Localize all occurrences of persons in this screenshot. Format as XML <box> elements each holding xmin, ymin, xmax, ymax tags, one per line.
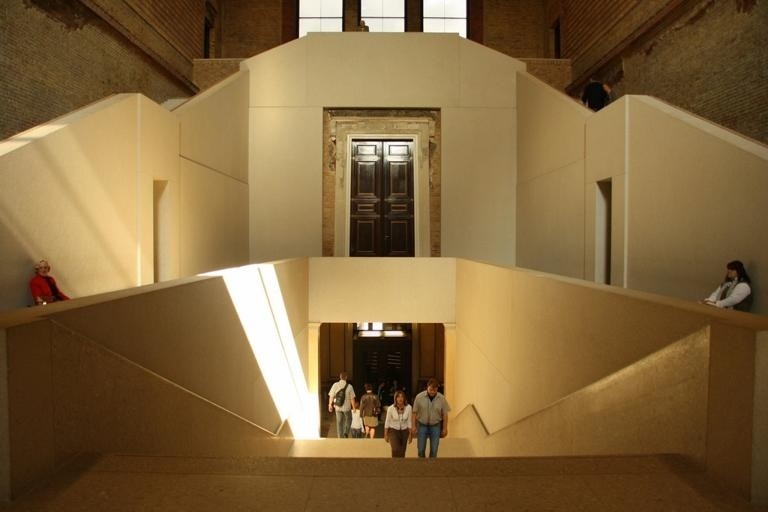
<box><xmin>704</xmin><ymin>260</ymin><xmax>753</xmax><ymax>312</ymax></box>
<box><xmin>328</xmin><ymin>371</ymin><xmax>405</xmax><ymax>439</ymax></box>
<box><xmin>412</xmin><ymin>378</ymin><xmax>451</xmax><ymax>458</ymax></box>
<box><xmin>356</xmin><ymin>21</ymin><xmax>369</xmax><ymax>32</ymax></box>
<box><xmin>29</xmin><ymin>258</ymin><xmax>70</xmax><ymax>306</ymax></box>
<box><xmin>581</xmin><ymin>74</ymin><xmax>610</xmax><ymax>112</ymax></box>
<box><xmin>384</xmin><ymin>391</ymin><xmax>412</xmax><ymax>457</ymax></box>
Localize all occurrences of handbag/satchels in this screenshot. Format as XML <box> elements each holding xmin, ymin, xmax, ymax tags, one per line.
<box><xmin>372</xmin><ymin>406</ymin><xmax>380</xmax><ymax>416</ymax></box>
<box><xmin>332</xmin><ymin>389</ymin><xmax>345</xmax><ymax>406</ymax></box>
